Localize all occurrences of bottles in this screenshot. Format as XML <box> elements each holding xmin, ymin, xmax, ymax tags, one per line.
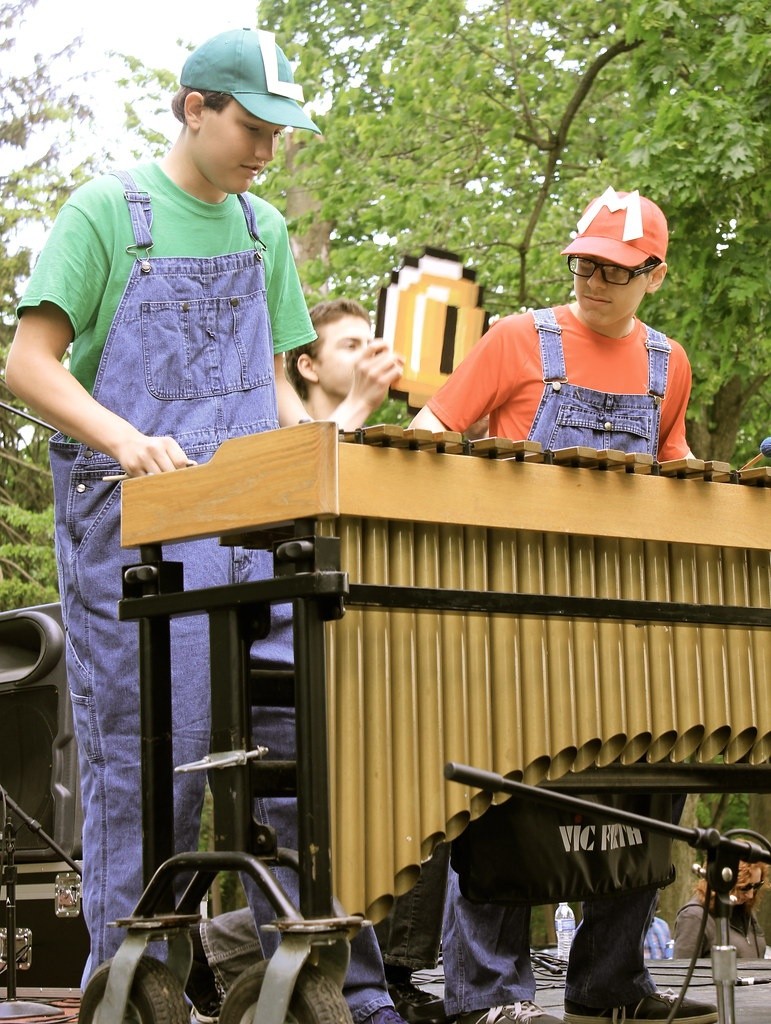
<box><xmin>554</xmin><ymin>903</ymin><xmax>575</xmax><ymax>961</ymax></box>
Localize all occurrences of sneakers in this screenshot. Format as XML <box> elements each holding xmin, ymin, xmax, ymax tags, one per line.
<box><xmin>363</xmin><ymin>1007</ymin><xmax>407</xmax><ymax>1024</ymax></box>
<box><xmin>564</xmin><ymin>987</ymin><xmax>719</xmax><ymax>1024</ymax></box>
<box><xmin>184</xmin><ymin>947</ymin><xmax>220</xmax><ymax>1023</ymax></box>
<box><xmin>387</xmin><ymin>982</ymin><xmax>451</xmax><ymax>1023</ymax></box>
<box><xmin>459</xmin><ymin>999</ymin><xmax>563</xmax><ymax>1024</ymax></box>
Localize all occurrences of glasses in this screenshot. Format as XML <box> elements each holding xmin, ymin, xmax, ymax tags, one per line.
<box><xmin>567</xmin><ymin>260</ymin><xmax>662</xmax><ymax>285</ymax></box>
<box><xmin>738</xmin><ymin>879</ymin><xmax>765</xmax><ymax>891</ymax></box>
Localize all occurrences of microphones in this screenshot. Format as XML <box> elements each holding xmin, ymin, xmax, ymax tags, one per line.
<box><xmin>760</xmin><ymin>437</ymin><xmax>771</xmax><ymax>458</ymax></box>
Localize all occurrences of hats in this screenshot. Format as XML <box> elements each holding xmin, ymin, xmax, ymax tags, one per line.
<box><xmin>181</xmin><ymin>27</ymin><xmax>322</xmax><ymax>136</ymax></box>
<box><xmin>560</xmin><ymin>185</ymin><xmax>668</xmax><ymax>265</ymax></box>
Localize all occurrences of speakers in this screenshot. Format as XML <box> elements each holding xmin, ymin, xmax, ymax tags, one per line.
<box><xmin>0</xmin><ymin>601</ymin><xmax>84</xmax><ymax>863</ymax></box>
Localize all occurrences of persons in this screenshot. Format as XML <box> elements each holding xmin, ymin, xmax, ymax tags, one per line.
<box><xmin>287</xmin><ymin>296</ymin><xmax>450</xmax><ymax>1024</ymax></box>
<box><xmin>6</xmin><ymin>28</ymin><xmax>406</xmax><ymax>1024</ymax></box>
<box><xmin>407</xmin><ymin>188</ymin><xmax>718</xmax><ymax>1024</ymax></box>
<box><xmin>644</xmin><ymin>838</ymin><xmax>768</xmax><ymax>960</ymax></box>
